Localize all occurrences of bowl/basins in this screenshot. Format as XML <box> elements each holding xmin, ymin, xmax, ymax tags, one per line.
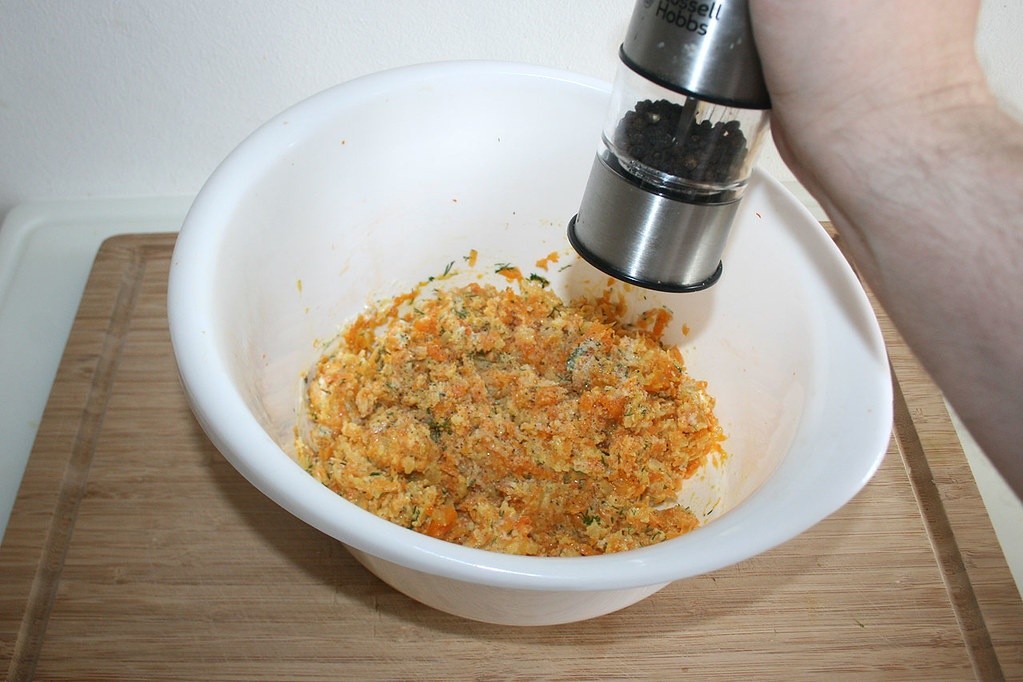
<box><xmin>169</xmin><ymin>54</ymin><xmax>893</xmax><ymax>631</ymax></box>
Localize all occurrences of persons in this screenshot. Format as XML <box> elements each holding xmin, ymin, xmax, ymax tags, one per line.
<box><xmin>750</xmin><ymin>0</ymin><xmax>1022</xmax><ymax>498</ymax></box>
